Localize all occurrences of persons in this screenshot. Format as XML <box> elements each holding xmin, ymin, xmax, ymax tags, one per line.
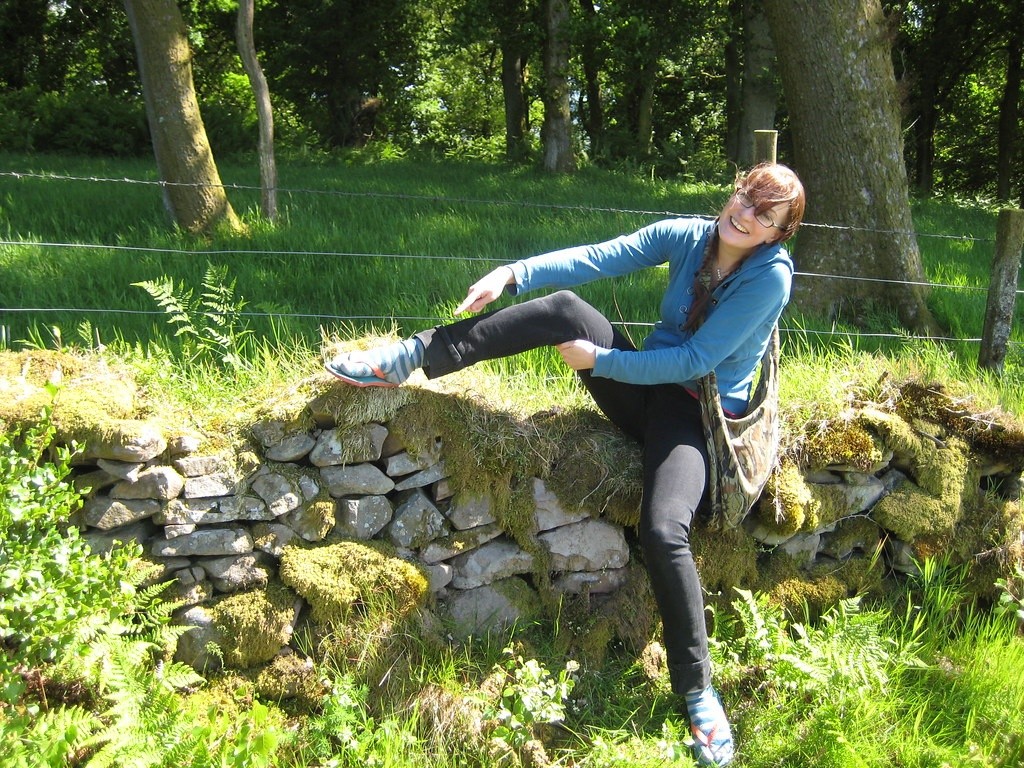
<box><xmin>325</xmin><ymin>162</ymin><xmax>805</xmax><ymax>768</ymax></box>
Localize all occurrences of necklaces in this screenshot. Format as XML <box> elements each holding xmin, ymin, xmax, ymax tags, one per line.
<box><xmin>716</xmin><ymin>264</ymin><xmax>723</xmax><ymax>280</ymax></box>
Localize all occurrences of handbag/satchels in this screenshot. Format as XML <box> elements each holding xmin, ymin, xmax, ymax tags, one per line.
<box><xmin>700</xmin><ymin>373</ymin><xmax>779</xmax><ymax>537</ymax></box>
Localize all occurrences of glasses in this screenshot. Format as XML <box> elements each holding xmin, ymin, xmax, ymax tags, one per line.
<box><xmin>735</xmin><ymin>187</ymin><xmax>787</xmax><ymax>232</ymax></box>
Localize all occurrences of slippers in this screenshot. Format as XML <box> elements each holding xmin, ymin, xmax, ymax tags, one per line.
<box><xmin>325</xmin><ymin>351</ymin><xmax>399</xmax><ymax>388</ymax></box>
<box><xmin>691</xmin><ymin>692</ymin><xmax>733</xmax><ymax>766</ymax></box>
